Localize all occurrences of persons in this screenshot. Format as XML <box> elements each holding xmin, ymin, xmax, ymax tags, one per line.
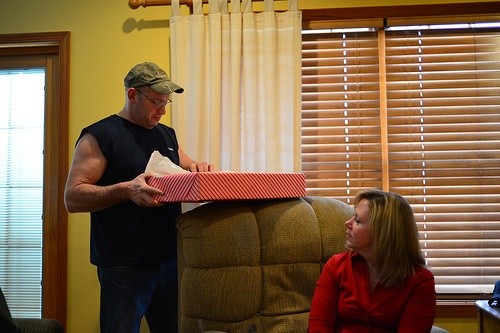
<box><xmin>63</xmin><ymin>62</ymin><xmax>215</xmax><ymax>333</ymax></box>
<box><xmin>307</xmin><ymin>191</ymin><xmax>436</xmax><ymax>333</ymax></box>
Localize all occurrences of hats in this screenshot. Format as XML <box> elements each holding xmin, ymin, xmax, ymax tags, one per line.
<box><xmin>124</xmin><ymin>62</ymin><xmax>183</xmax><ymax>95</ymax></box>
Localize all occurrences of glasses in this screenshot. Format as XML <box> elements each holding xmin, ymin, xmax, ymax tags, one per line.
<box><xmin>133</xmin><ymin>87</ymin><xmax>172</xmax><ymax>107</ymax></box>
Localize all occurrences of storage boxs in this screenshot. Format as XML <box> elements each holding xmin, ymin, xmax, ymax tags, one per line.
<box><xmin>146</xmin><ymin>171</ymin><xmax>307</xmax><ymax>203</ymax></box>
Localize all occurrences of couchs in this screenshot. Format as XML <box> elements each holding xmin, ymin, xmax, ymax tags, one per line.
<box><xmin>176</xmin><ymin>196</ymin><xmax>449</xmax><ymax>333</ymax></box>
